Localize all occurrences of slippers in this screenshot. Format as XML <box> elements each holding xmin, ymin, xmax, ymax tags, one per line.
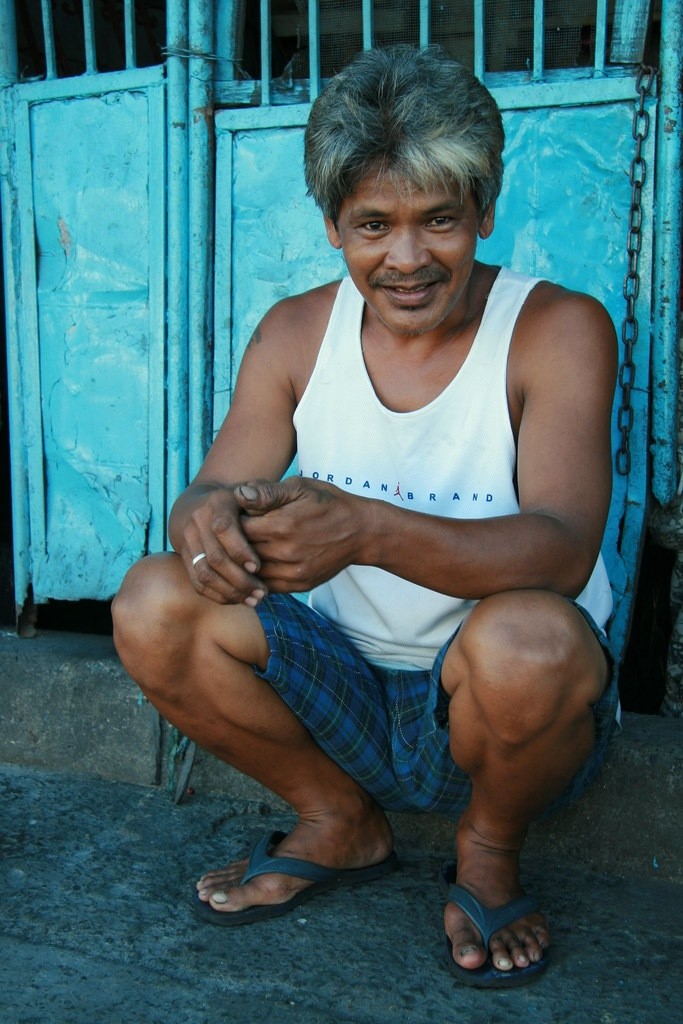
<box><xmin>185</xmin><ymin>827</ymin><xmax>550</xmax><ymax>987</ymax></box>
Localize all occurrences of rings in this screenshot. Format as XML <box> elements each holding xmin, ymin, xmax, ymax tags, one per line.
<box><xmin>191</xmin><ymin>553</ymin><xmax>206</xmax><ymax>567</ymax></box>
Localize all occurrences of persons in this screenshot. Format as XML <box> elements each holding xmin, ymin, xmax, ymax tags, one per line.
<box><xmin>109</xmin><ymin>40</ymin><xmax>620</xmax><ymax>992</ymax></box>
